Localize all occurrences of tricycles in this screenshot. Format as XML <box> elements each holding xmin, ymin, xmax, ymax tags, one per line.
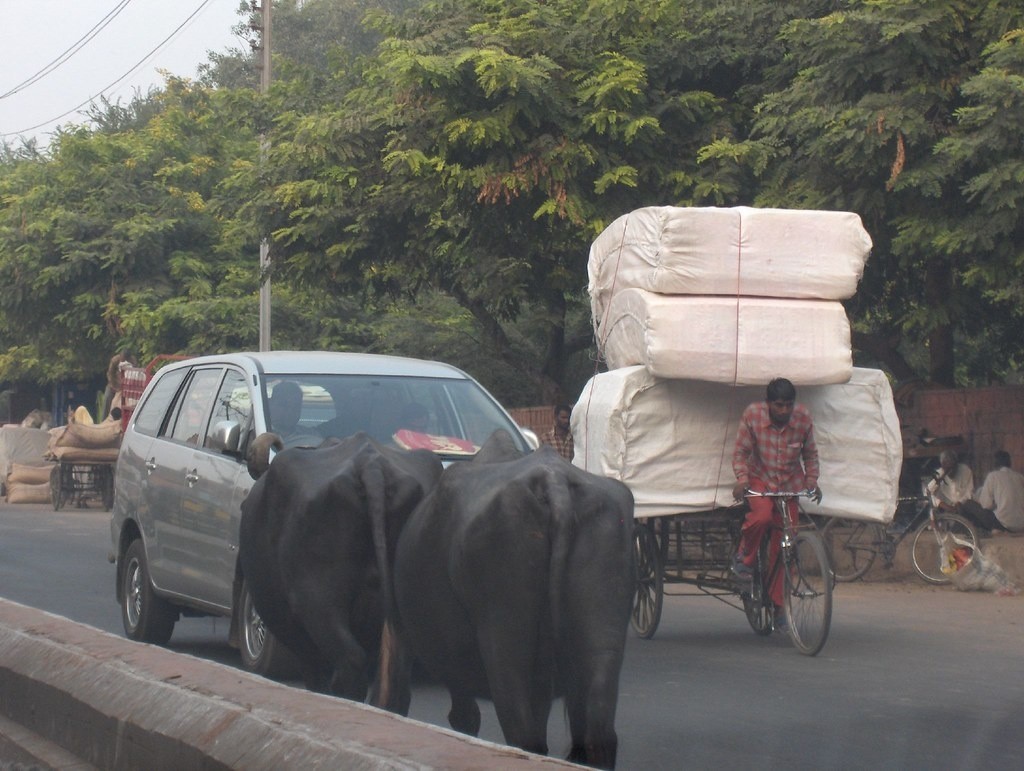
<box><xmin>627</xmin><ymin>486</ymin><xmax>834</xmax><ymax>656</ymax></box>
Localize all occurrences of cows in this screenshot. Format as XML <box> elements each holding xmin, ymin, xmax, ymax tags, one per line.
<box><xmin>388</xmin><ymin>425</ymin><xmax>637</xmax><ymax>771</ymax></box>
<box><xmin>235</xmin><ymin>431</ymin><xmax>447</xmax><ymax>718</ymax></box>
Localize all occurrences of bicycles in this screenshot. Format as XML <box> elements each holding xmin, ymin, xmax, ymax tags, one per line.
<box><xmin>817</xmin><ymin>471</ymin><xmax>981</xmax><ymax>584</ymax></box>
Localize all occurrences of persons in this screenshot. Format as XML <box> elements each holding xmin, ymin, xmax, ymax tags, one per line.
<box><xmin>270</xmin><ymin>379</ymin><xmax>304</xmax><ymax>435</ymax></box>
<box><xmin>731</xmin><ymin>378</ymin><xmax>822</xmax><ymax>627</ymax></box>
<box><xmin>924</xmin><ymin>449</ymin><xmax>1024</xmax><ymax>534</ymax></box>
<box><xmin>537</xmin><ymin>403</ymin><xmax>574</xmax><ymax>462</ymax></box>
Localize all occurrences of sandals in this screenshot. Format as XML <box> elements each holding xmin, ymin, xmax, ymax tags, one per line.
<box><xmin>769</xmin><ymin>606</ymin><xmax>788</xmax><ymax>632</ymax></box>
<box><xmin>729</xmin><ymin>556</ymin><xmax>754</xmax><ymax>580</ymax></box>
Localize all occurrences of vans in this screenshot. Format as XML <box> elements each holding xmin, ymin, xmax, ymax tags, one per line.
<box><xmin>107</xmin><ymin>349</ymin><xmax>542</xmax><ymax>681</ymax></box>
